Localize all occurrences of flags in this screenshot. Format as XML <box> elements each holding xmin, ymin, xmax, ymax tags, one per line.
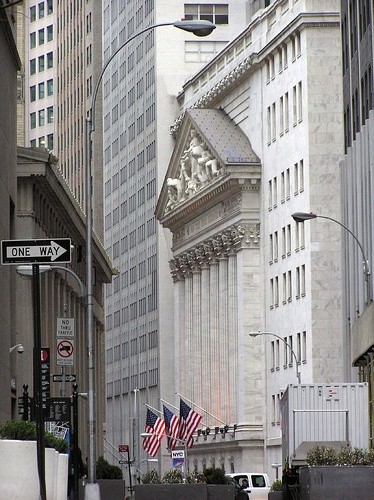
<box><xmin>142</xmin><ymin>408</ymin><xmax>165</xmax><ymax>457</ymax></box>
<box><xmin>179</xmin><ymin>399</ymin><xmax>203</xmax><ymax>448</ymax></box>
<box><xmin>163</xmin><ymin>405</ymin><xmax>180</xmax><ymax>454</ymax></box>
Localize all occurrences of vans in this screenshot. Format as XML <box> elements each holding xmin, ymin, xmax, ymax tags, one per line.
<box><xmin>227</xmin><ymin>473</ymin><xmax>269</xmax><ymax>500</ymax></box>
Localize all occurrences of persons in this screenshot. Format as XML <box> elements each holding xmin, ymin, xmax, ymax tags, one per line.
<box><xmin>162</xmin><ymin>129</ymin><xmax>223</xmax><ymax>208</ymax></box>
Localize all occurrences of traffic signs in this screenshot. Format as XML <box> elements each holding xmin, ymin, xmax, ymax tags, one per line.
<box><xmin>1</xmin><ymin>238</ymin><xmax>72</xmax><ymax>266</ymax></box>
<box><xmin>53</xmin><ymin>375</ymin><xmax>76</xmax><ymax>383</ymax></box>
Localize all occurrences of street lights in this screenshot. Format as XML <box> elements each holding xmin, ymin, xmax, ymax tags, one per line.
<box><xmin>84</xmin><ymin>17</ymin><xmax>216</xmax><ymax>500</ymax></box>
<box><xmin>134</xmin><ymin>458</ymin><xmax>160</xmax><ymax>486</ymax></box>
<box><xmin>140</xmin><ymin>433</ymin><xmax>188</xmax><ymax>484</ymax></box>
<box><xmin>249</xmin><ymin>332</ymin><xmax>301</xmax><ymax>383</ymax></box>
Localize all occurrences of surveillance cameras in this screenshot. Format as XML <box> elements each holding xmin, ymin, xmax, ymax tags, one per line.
<box><xmin>17</xmin><ymin>346</ymin><xmax>24</xmax><ymax>354</ymax></box>
